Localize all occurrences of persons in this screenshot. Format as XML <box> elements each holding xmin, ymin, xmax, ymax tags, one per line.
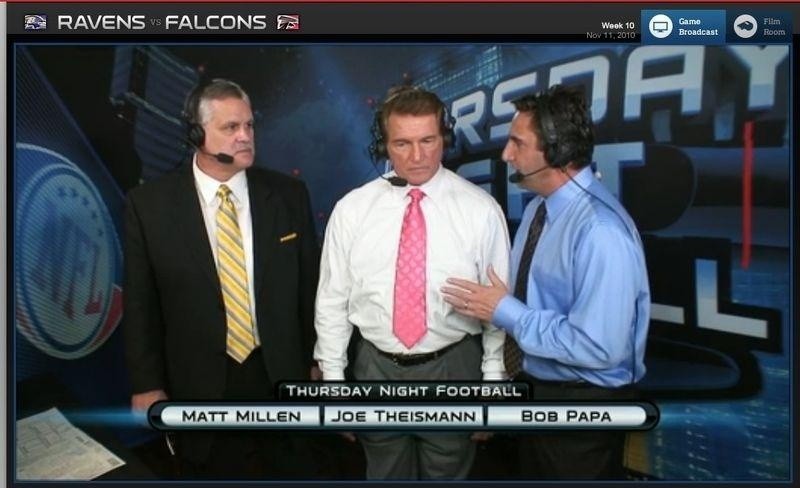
<box><xmin>313</xmin><ymin>85</ymin><xmax>510</xmax><ymax>480</ymax></box>
<box><xmin>440</xmin><ymin>85</ymin><xmax>650</xmax><ymax>479</ymax></box>
<box><xmin>121</xmin><ymin>79</ymin><xmax>322</xmax><ymax>478</ymax></box>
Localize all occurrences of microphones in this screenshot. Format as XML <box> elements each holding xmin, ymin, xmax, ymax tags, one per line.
<box><xmin>187</xmin><ymin>136</ymin><xmax>233</xmax><ymax>163</ymax></box>
<box><xmin>509</xmin><ymin>149</ymin><xmax>562</xmax><ymax>182</ymax></box>
<box><xmin>368</xmin><ymin>145</ymin><xmax>408</xmax><ymax>186</ymax></box>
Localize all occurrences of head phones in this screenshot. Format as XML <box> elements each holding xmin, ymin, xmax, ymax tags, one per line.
<box><xmin>370</xmin><ymin>89</ymin><xmax>456</xmax><ymax>162</ymax></box>
<box><xmin>182</xmin><ymin>78</ymin><xmax>222</xmax><ymax>149</ymax></box>
<box><xmin>535</xmin><ymin>89</ymin><xmax>572</xmax><ymax>169</ymax></box>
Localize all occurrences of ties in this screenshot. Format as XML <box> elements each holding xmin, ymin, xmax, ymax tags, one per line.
<box><xmin>393</xmin><ymin>189</ymin><xmax>428</xmax><ymax>349</ymax></box>
<box><xmin>503</xmin><ymin>200</ymin><xmax>547</xmax><ymax>377</ymax></box>
<box><xmin>215</xmin><ymin>184</ymin><xmax>256</xmax><ymax>366</ymax></box>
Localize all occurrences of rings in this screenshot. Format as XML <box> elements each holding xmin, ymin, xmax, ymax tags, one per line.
<box><xmin>464</xmin><ymin>303</ymin><xmax>468</xmax><ymax>310</ymax></box>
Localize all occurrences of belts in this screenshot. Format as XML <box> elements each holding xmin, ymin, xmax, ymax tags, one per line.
<box><xmin>514</xmin><ymin>372</ymin><xmax>591</xmax><ymax>389</ymax></box>
<box><xmin>374</xmin><ymin>333</ymin><xmax>469</xmax><ymax>367</ymax></box>
